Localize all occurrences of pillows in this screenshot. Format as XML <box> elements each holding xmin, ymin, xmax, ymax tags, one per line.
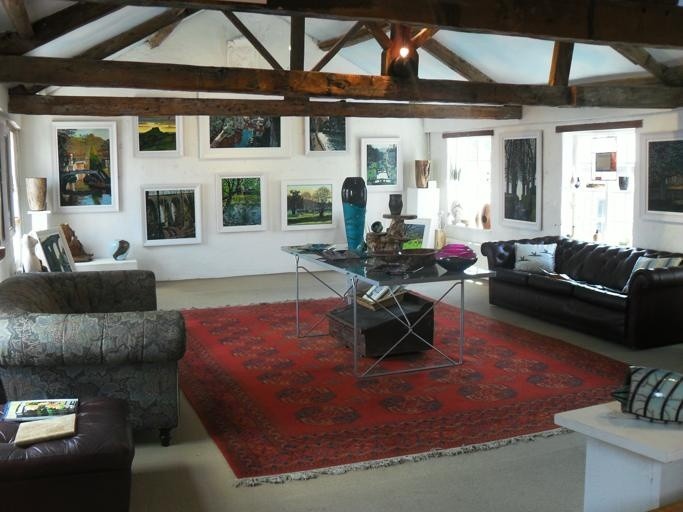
<box><xmin>623</xmin><ymin>256</ymin><xmax>681</xmax><ymax>294</ymax></box>
<box><xmin>512</xmin><ymin>239</ymin><xmax>558</xmax><ymax>277</ymax></box>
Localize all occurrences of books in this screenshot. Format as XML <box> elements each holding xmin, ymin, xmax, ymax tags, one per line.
<box><xmin>0</xmin><ymin>398</ymin><xmax>81</xmax><ymax>423</ymax></box>
<box><xmin>13</xmin><ymin>412</ymin><xmax>77</xmax><ymax>445</ymax></box>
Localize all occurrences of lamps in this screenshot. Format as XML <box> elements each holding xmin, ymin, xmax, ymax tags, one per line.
<box><xmin>394</xmin><ymin>36</ymin><xmax>417</xmax><ymax>62</ymax></box>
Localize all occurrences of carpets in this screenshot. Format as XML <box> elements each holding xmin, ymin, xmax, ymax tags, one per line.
<box><xmin>149</xmin><ymin>288</ymin><xmax>640</xmax><ymax>491</ymax></box>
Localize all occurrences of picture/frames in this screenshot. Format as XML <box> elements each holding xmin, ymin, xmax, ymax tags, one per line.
<box><xmin>214</xmin><ymin>172</ymin><xmax>268</xmax><ymax>234</ymax></box>
<box><xmin>638</xmin><ymin>129</ymin><xmax>683</xmax><ymax>226</ymax></box>
<box><xmin>45</xmin><ymin>115</ymin><xmax>203</xmax><ymax>249</ymax></box>
<box><xmin>197</xmin><ymin>115</ymin><xmax>293</xmax><ymax>161</ymax></box>
<box><xmin>278</xmin><ymin>175</ymin><xmax>340</xmax><ymax>232</ymax></box>
<box><xmin>497</xmin><ymin>131</ymin><xmax>545</xmax><ymax>231</ymax></box>
<box><xmin>358</xmin><ymin>134</ymin><xmax>405</xmax><ymax>194</ymax></box>
<box><xmin>301</xmin><ymin>114</ymin><xmax>354</xmax><ymax>157</ymax></box>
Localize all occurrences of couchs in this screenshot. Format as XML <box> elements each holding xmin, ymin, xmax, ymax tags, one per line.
<box><xmin>0</xmin><ymin>266</ymin><xmax>187</xmax><ymax>446</ymax></box>
<box><xmin>480</xmin><ymin>233</ymin><xmax>681</xmax><ymax>352</ymax></box>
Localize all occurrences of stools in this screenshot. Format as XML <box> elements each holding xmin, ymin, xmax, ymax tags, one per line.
<box><xmin>0</xmin><ymin>393</ymin><xmax>138</xmax><ymax>512</ymax></box>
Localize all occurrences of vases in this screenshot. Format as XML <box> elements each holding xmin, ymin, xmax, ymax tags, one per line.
<box><xmin>341</xmin><ymin>175</ymin><xmax>367</xmax><ymax>252</ymax></box>
<box><xmin>387</xmin><ymin>193</ymin><xmax>403</xmax><ymax>216</ymax></box>
<box><xmin>22</xmin><ymin>174</ymin><xmax>49</xmax><ymax>211</ymax></box>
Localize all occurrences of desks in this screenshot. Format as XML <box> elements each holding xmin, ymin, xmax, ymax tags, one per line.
<box><xmin>24</xmin><ymin>211</ymin><xmax>53</xmax><ymax>233</ymax></box>
<box><xmin>280</xmin><ymin>243</ymin><xmax>497</xmax><ymax>380</ymax></box>
<box><xmin>548</xmin><ymin>400</ymin><xmax>682</xmax><ymax>511</ymax></box>
<box><xmin>380</xmin><ymin>213</ymin><xmax>417</xmax><ymax>228</ymax></box>
